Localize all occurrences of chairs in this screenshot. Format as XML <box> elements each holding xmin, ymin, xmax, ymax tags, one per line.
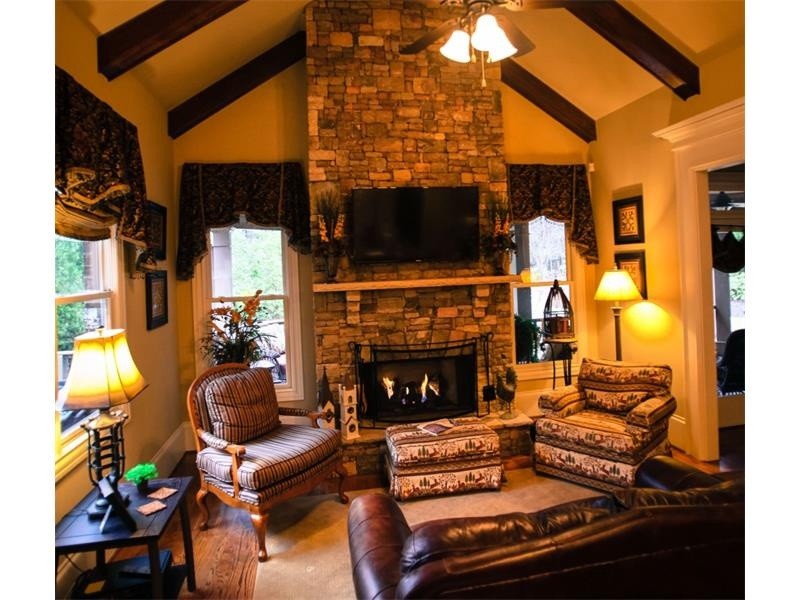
<box><xmin>716</xmin><ymin>327</ymin><xmax>745</xmax><ymax>391</ymax></box>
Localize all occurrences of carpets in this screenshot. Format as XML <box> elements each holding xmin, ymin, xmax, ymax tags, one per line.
<box><xmin>252</xmin><ymin>461</ymin><xmax>609</xmax><ymax>600</ymax></box>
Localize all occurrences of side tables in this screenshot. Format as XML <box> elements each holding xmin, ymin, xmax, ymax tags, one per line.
<box><xmin>55</xmin><ymin>475</ymin><xmax>197</xmax><ymax>600</ymax></box>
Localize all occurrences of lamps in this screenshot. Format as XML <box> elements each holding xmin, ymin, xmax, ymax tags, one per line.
<box><xmin>439</xmin><ymin>6</ymin><xmax>517</xmax><ymax>62</ymax></box>
<box><xmin>57</xmin><ymin>326</ymin><xmax>149</xmax><ymax>521</ymax></box>
<box><xmin>592</xmin><ymin>269</ymin><xmax>644</xmax><ymax>362</ymax></box>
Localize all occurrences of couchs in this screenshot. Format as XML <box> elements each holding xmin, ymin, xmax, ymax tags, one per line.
<box><xmin>345</xmin><ymin>454</ymin><xmax>746</xmax><ymax>600</ymax></box>
<box><xmin>534</xmin><ymin>358</ymin><xmax>676</xmax><ymax>492</ymax></box>
<box><xmin>185</xmin><ymin>362</ymin><xmax>349</xmax><ymax>563</ymax></box>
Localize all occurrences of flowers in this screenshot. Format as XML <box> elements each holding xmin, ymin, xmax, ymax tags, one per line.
<box><xmin>200</xmin><ymin>291</ymin><xmax>277</xmax><ymax>364</ymax></box>
<box><xmin>315</xmin><ymin>188</ymin><xmax>344</xmax><ymax>255</ymax></box>
<box><xmin>484</xmin><ymin>194</ymin><xmax>517</xmax><ymax>257</ymax></box>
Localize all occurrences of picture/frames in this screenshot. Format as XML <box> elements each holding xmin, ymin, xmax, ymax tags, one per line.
<box><xmin>614</xmin><ymin>250</ymin><xmax>646</xmax><ymax>300</ymax></box>
<box><xmin>612</xmin><ymin>196</ymin><xmax>644</xmax><ymax>244</ymax></box>
<box><xmin>146</xmin><ymin>270</ymin><xmax>168</xmax><ymax>329</ymax></box>
<box><xmin>146</xmin><ymin>200</ymin><xmax>167</xmax><ymax>261</ymax></box>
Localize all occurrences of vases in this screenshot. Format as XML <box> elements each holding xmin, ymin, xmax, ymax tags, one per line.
<box><xmin>492</xmin><ymin>249</ymin><xmax>505</xmax><ymax>274</ymax></box>
<box><xmin>324</xmin><ymin>255</ymin><xmax>340</xmax><ymax>284</ymax></box>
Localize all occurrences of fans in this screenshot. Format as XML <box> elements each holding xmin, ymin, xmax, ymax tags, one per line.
<box><xmin>399</xmin><ymin>0</ymin><xmax>536</xmax><ymax>57</ymax></box>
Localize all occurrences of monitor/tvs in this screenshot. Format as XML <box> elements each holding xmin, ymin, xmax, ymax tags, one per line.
<box><xmin>351</xmin><ymin>186</ymin><xmax>480</xmax><ymax>263</ymax></box>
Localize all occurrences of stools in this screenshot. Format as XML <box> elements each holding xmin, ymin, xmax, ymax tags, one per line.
<box><xmin>385</xmin><ymin>416</ymin><xmax>507</xmax><ymax>501</ymax></box>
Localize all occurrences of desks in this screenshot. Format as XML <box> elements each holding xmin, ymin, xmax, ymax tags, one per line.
<box><xmin>541</xmin><ymin>338</ymin><xmax>579</xmax><ymax>389</ymax></box>
